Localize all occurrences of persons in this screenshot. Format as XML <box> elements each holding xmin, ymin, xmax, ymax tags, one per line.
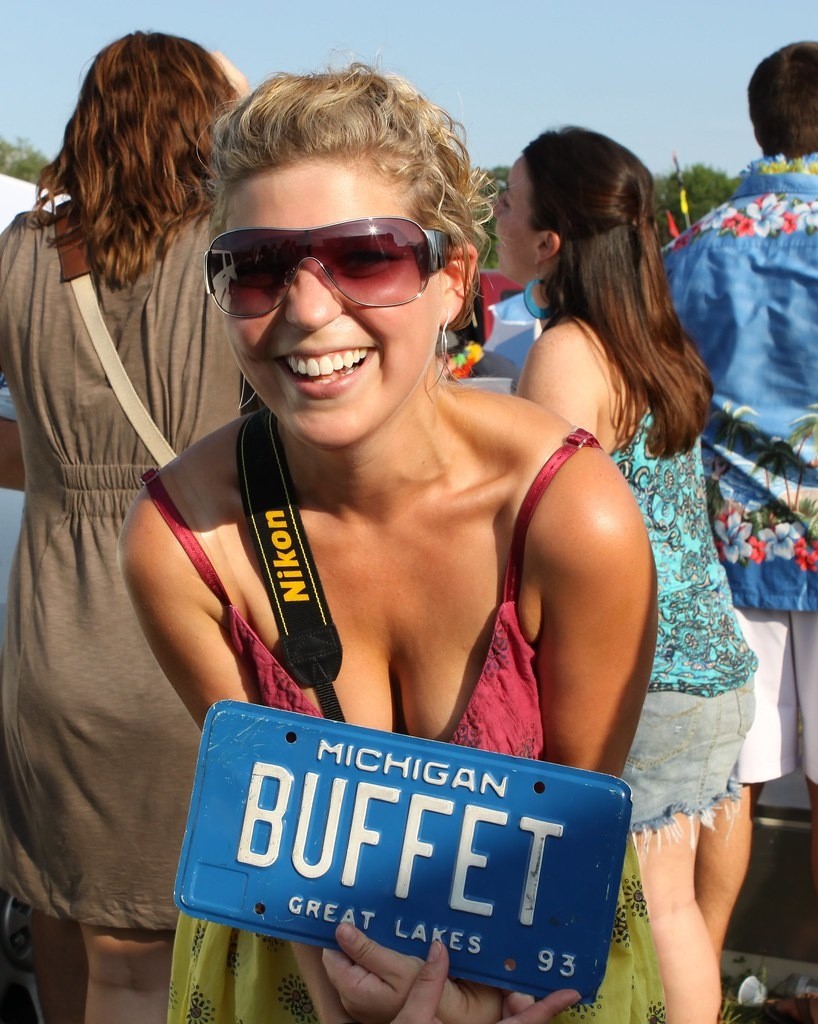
<box><xmin>0</xmin><ymin>31</ymin><xmax>267</xmax><ymax>1024</ymax></box>
<box><xmin>116</xmin><ymin>64</ymin><xmax>666</xmax><ymax>1024</ymax></box>
<box><xmin>659</xmin><ymin>41</ymin><xmax>818</xmax><ymax>967</ymax></box>
<box><xmin>490</xmin><ymin>125</ymin><xmax>759</xmax><ymax>1024</ymax></box>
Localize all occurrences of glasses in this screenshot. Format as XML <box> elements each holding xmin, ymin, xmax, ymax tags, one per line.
<box><xmin>203</xmin><ymin>217</ymin><xmax>456</xmax><ymax>319</ymax></box>
